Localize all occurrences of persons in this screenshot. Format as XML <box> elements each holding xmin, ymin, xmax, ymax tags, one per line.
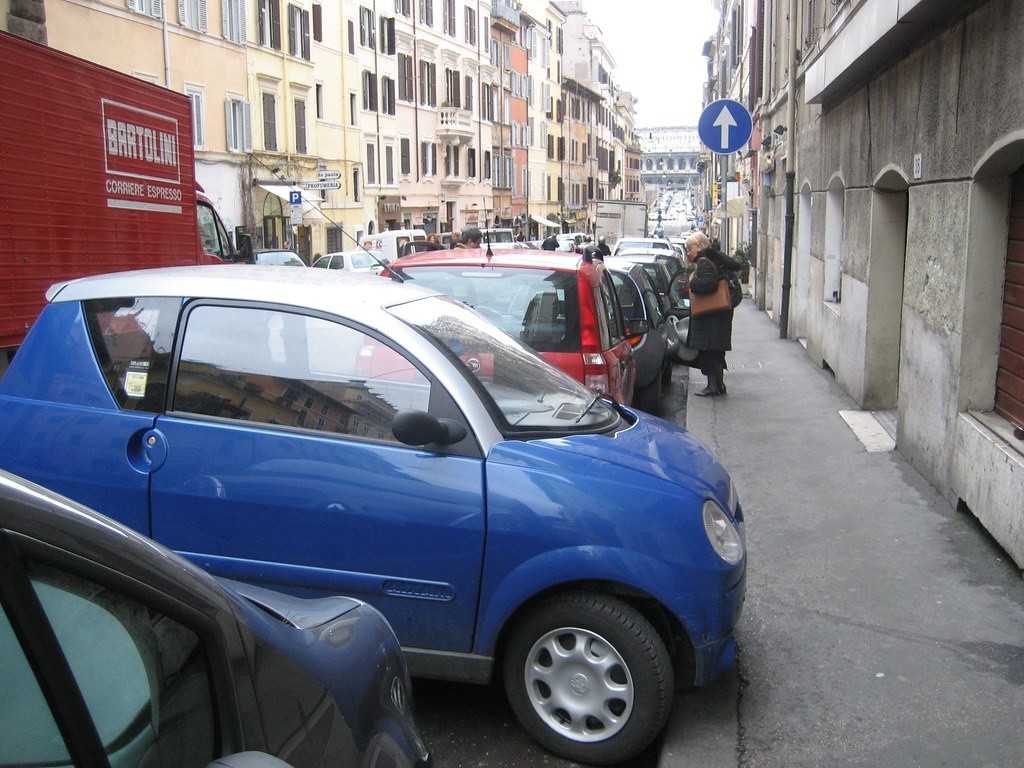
<box><xmin>651</xmin><ymin>223</ymin><xmax>743</xmax><ymax>397</ymax></box>
<box><xmin>428</xmin><ymin>229</ymin><xmax>609</xmax><ymax>262</ymax></box>
<box><xmin>282</xmin><ymin>241</ymin><xmax>298</xmax><ymax>251</ymax></box>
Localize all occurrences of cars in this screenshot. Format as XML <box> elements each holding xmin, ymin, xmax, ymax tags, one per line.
<box><xmin>0</xmin><ymin>263</ymin><xmax>748</xmax><ymax>767</ymax></box>
<box><xmin>1</xmin><ymin>463</ymin><xmax>433</xmax><ymax>768</ymax></box>
<box><xmin>248</xmin><ymin>230</ymin><xmax>687</xmax><ymax>429</ymax></box>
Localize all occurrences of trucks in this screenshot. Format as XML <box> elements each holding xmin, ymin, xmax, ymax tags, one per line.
<box><xmin>0</xmin><ymin>27</ymin><xmax>258</xmax><ymax>375</ymax></box>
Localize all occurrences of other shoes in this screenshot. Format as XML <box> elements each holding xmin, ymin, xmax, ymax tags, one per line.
<box><xmin>720</xmin><ymin>384</ymin><xmax>726</xmax><ymax>393</ymax></box>
<box><xmin>694</xmin><ymin>387</ymin><xmax>719</xmax><ymax>395</ymax></box>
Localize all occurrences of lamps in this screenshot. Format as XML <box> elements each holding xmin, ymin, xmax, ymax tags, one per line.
<box><xmin>761</xmin><ymin>136</ymin><xmax>771</xmax><ymax>147</ymax></box>
<box><xmin>774</xmin><ymin>125</ymin><xmax>787</xmax><ymax>134</ymax></box>
<box><xmin>746</xmin><ymin>149</ymin><xmax>757</xmax><ymax>156</ymax></box>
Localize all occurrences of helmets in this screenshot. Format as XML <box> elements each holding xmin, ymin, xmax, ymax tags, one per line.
<box><xmin>584</xmin><ymin>236</ymin><xmax>591</xmax><ymax>242</ymax></box>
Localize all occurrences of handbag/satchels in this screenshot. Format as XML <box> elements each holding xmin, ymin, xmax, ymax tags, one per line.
<box><xmin>689</xmin><ymin>257</ymin><xmax>732</xmax><ymax>316</ymax></box>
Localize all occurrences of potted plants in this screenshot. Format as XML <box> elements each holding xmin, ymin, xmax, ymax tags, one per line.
<box><xmin>609</xmin><ymin>169</ymin><xmax>621</xmax><ymax>189</ymax></box>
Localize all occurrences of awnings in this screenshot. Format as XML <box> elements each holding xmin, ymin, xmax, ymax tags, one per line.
<box><xmin>714</xmin><ymin>194</ymin><xmax>745</xmax><ymax>219</ymax></box>
<box><xmin>253</xmin><ymin>183</ymin><xmax>325</xmax><ymax>217</ymax></box>
<box><xmin>532</xmin><ymin>215</ymin><xmax>578</xmax><ymax>229</ymax></box>
